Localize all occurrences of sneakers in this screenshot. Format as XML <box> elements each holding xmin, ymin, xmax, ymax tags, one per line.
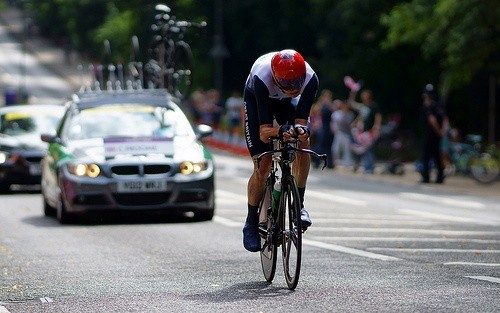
<box><xmin>291</xmin><ymin>205</ymin><xmax>312</xmax><ymax>226</ymax></box>
<box><xmin>243</xmin><ymin>220</ymin><xmax>261</xmax><ymax>252</ymax></box>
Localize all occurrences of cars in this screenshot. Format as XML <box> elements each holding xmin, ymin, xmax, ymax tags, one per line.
<box><xmin>40</xmin><ymin>91</ymin><xmax>216</xmax><ymax>223</ymax></box>
<box><xmin>0</xmin><ymin>102</ymin><xmax>64</xmax><ymax>196</ymax></box>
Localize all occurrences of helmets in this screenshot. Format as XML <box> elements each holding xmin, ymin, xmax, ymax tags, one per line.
<box><xmin>271</xmin><ymin>49</ymin><xmax>306</xmax><ymax>80</ymax></box>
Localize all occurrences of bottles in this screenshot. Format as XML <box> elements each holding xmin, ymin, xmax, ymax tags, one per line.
<box><xmin>272</xmin><ymin>181</ymin><xmax>283</xmax><ymax>207</ymax></box>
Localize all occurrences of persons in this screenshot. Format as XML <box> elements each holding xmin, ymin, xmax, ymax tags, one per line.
<box><xmin>241</xmin><ymin>48</ymin><xmax>320</xmax><ymax>252</ymax></box>
<box><xmin>190</xmin><ymin>84</ymin><xmax>456</xmax><ymax>184</ymax></box>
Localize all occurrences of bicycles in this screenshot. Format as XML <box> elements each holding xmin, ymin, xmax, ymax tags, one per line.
<box><xmin>414</xmin><ymin>129</ymin><xmax>496</xmax><ymax>188</ymax></box>
<box><xmin>140</xmin><ymin>6</ymin><xmax>209</xmax><ymax>101</ymax></box>
<box><xmin>257</xmin><ymin>133</ymin><xmax>328</xmax><ymax>288</ymax></box>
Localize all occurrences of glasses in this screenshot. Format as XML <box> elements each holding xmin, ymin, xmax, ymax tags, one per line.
<box><xmin>274</xmin><ymin>72</ymin><xmax>306</xmax><ymax>90</ymax></box>
